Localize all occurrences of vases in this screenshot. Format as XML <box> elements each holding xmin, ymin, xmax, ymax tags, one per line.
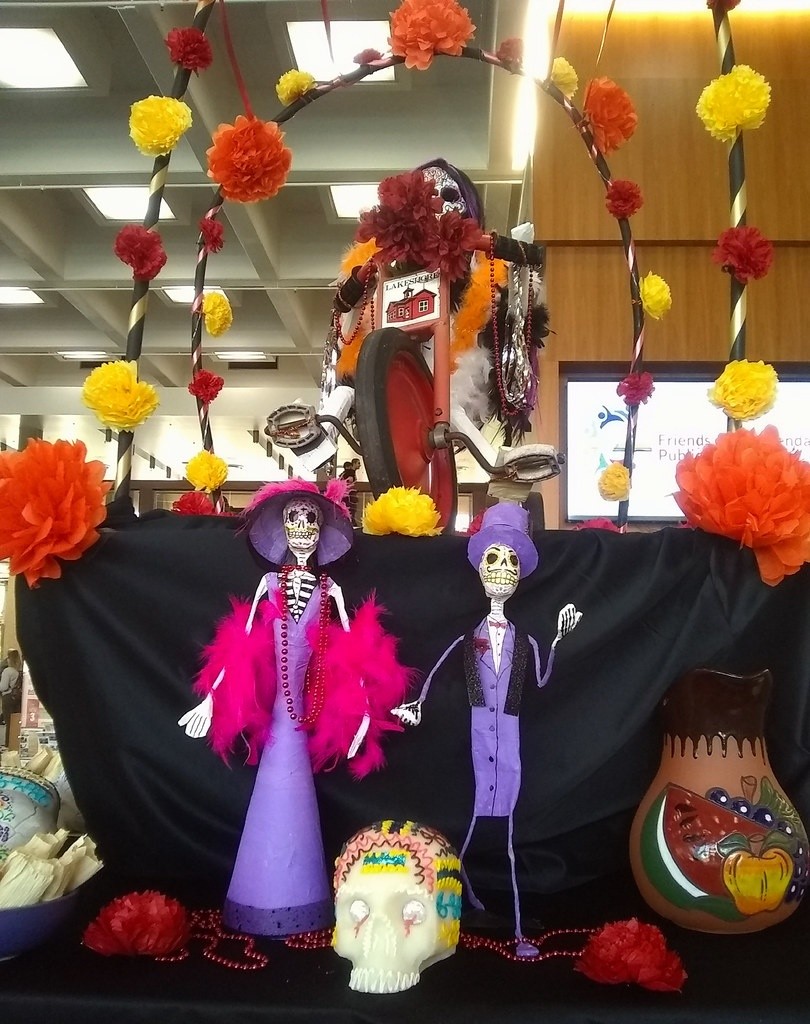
<box><xmin>631</xmin><ymin>666</ymin><xmax>809</xmax><ymax>933</ymax></box>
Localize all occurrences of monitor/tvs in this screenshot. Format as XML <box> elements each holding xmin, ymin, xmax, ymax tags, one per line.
<box><xmin>556</xmin><ymin>372</ymin><xmax>810</xmax><ymax>522</ymax></box>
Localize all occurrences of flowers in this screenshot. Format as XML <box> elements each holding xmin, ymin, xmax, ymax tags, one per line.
<box><xmin>84</xmin><ymin>890</ymin><xmax>189</xmax><ymax>955</ymax></box>
<box><xmin>696</xmin><ymin>65</ymin><xmax>771</xmax><ymax>143</ymax></box>
<box><xmin>362</xmin><ymin>486</ymin><xmax>444</xmax><ymax>538</ymax></box>
<box><xmin>82</xmin><ymin>360</ymin><xmax>159</xmax><ymax>433</ymax></box>
<box><xmin>203</xmin><ymin>292</ymin><xmax>232</xmax><ymax>339</ymax></box>
<box><xmin>0</xmin><ymin>437</ymin><xmax>113</xmax><ymax>590</ymax></box>
<box><xmin>606</xmin><ymin>178</ymin><xmax>643</xmax><ymax>220</ymax></box>
<box><xmin>353</xmin><ymin>0</ymin><xmax>638</xmax><ymax>155</ymax></box>
<box><xmin>714</xmin><ymin>224</ymin><xmax>775</xmax><ymax>286</ymax></box>
<box><xmin>189</xmin><ymin>369</ymin><xmax>225</xmax><ymax>404</ymax></box>
<box><xmin>113</xmin><ymin>224</ymin><xmax>168</xmax><ymax>281</ymax></box>
<box><xmin>171</xmin><ymin>491</ymin><xmax>217</xmax><ymax>516</ymax></box>
<box><xmin>574</xmin><ymin>358</ymin><xmax>810</xmax><ymax>587</ymax></box>
<box><xmin>198</xmin><ymin>216</ymin><xmax>225</xmax><ymax>254</ymax></box>
<box><xmin>576</xmin><ymin>921</ymin><xmax>688</xmax><ymax>990</ymax></box>
<box><xmin>186</xmin><ymin>450</ymin><xmax>228</xmax><ymax>493</ymax></box>
<box><xmin>354</xmin><ymin>170</ymin><xmax>484</xmax><ymax>281</ymax></box>
<box><xmin>128</xmin><ymin>27</ymin><xmax>316</xmax><ymax>204</ymax></box>
<box><xmin>639</xmin><ymin>269</ymin><xmax>672</xmax><ymax>320</ymax></box>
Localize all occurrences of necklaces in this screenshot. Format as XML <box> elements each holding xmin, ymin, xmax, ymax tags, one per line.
<box><xmin>280</xmin><ymin>566</ymin><xmax>327</xmax><ymax>723</ymax></box>
<box><xmin>284</xmin><ymin>928</ymin><xmax>333</xmax><ymax>949</ymax></box>
<box><xmin>458</xmin><ymin>928</ymin><xmax>601</xmax><ymax>960</ymax></box>
<box><xmin>155</xmin><ymin>908</ymin><xmax>268</xmax><ymax>970</ymax></box>
